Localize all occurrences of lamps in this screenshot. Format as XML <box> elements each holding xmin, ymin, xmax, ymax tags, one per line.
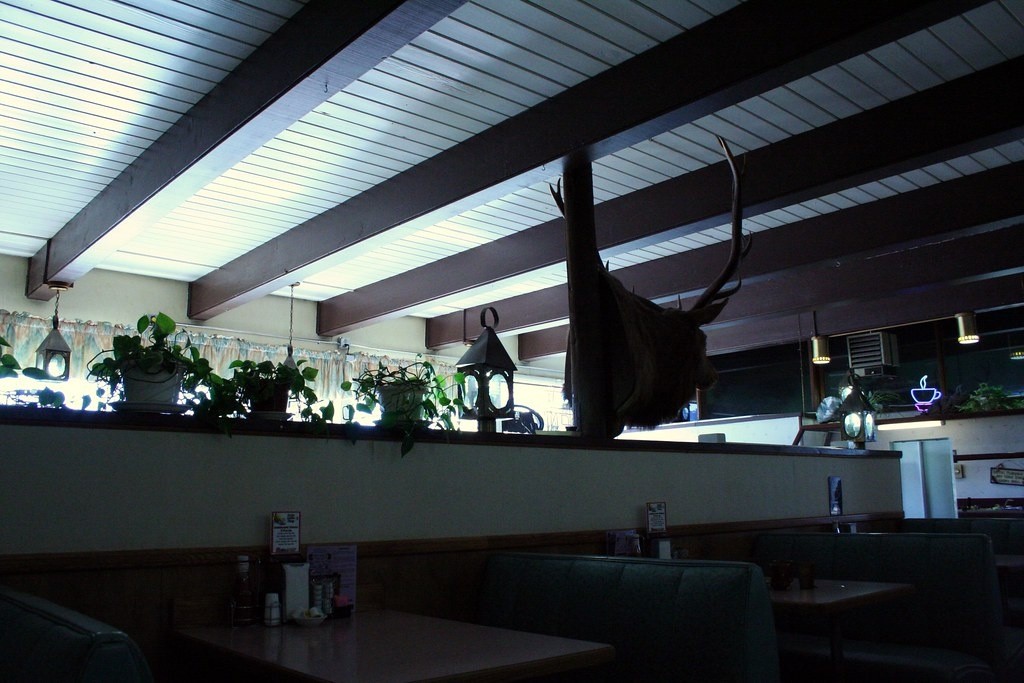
<box><xmin>839</xmin><ymin>374</ymin><xmax>878</xmax><ymax>449</ymax></box>
<box><xmin>455</xmin><ymin>305</ymin><xmax>516</xmax><ymax>431</ymax></box>
<box><xmin>811</xmin><ymin>336</ymin><xmax>830</xmax><ymax>364</ymax></box>
<box><xmin>280</xmin><ymin>282</ymin><xmax>300</xmax><ymax>402</ymax></box>
<box><xmin>35</xmin><ymin>285</ymin><xmax>71</xmax><ymax>383</ymax></box>
<box><xmin>954</xmin><ymin>311</ymin><xmax>980</xmax><ymax>345</ymax></box>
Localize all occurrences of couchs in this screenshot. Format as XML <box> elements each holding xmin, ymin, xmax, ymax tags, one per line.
<box><xmin>0</xmin><ymin>517</ymin><xmax>1024</xmax><ymax>683</ymax></box>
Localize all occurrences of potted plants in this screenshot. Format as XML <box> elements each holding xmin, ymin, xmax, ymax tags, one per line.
<box><xmin>340</xmin><ymin>352</ymin><xmax>465</xmax><ymax>431</ymax></box>
<box><xmin>81</xmin><ymin>311</ymin><xmax>335</xmax><ymax>424</ymax></box>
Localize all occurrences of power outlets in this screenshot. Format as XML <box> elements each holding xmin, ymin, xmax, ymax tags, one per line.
<box><xmin>338</xmin><ymin>337</ymin><xmax>350</xmax><ymax>350</ymax></box>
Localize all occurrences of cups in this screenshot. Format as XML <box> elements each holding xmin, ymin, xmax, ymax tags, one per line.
<box><xmin>796</xmin><ymin>559</ymin><xmax>816</xmax><ymax>590</ymax></box>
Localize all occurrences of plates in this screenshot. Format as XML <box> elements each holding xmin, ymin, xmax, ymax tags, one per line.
<box><xmin>290</xmin><ymin>613</ymin><xmax>328</xmax><ymax>627</ymax></box>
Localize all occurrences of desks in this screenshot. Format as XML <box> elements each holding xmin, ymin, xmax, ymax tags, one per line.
<box><xmin>764</xmin><ymin>577</ymin><xmax>916</xmax><ymax>683</ymax></box>
<box><xmin>170</xmin><ymin>607</ymin><xmax>617</xmax><ymax>683</ymax></box>
<box><xmin>993</xmin><ymin>552</ymin><xmax>1024</xmax><ymax>627</ymax></box>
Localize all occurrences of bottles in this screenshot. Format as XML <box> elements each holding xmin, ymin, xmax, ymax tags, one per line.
<box><xmin>265</xmin><ymin>593</ymin><xmax>281</xmax><ymax>626</ymax></box>
<box><xmin>831</xmin><ymin>520</ymin><xmax>840</xmax><ymax>533</ymax></box>
<box><xmin>630</xmin><ymin>534</ymin><xmax>643</xmax><ymax>557</ymax></box>
<box><xmin>227</xmin><ymin>554</ymin><xmax>260</xmax><ymax>629</ymax></box>
